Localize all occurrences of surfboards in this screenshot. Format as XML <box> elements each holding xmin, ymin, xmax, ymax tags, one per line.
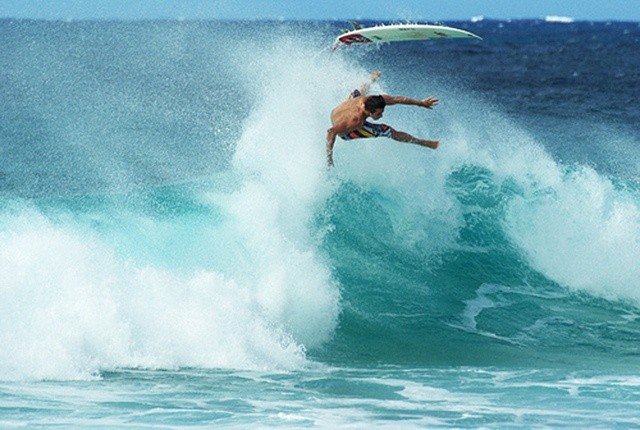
<box><xmin>336</xmin><ymin>20</ymin><xmax>483</xmax><ymax>46</ymax></box>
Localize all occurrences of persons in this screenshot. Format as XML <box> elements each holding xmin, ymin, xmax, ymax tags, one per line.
<box><xmin>326</xmin><ymin>70</ymin><xmax>440</xmax><ymax>167</ymax></box>
